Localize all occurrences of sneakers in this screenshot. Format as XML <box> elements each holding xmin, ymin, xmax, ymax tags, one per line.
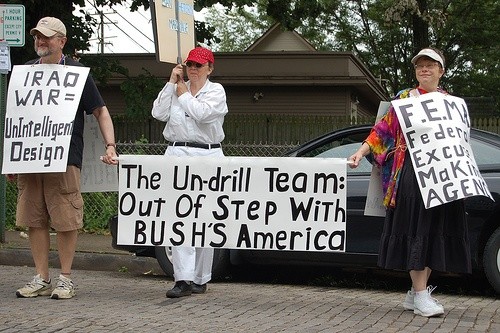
<box><xmin>192</xmin><ymin>282</ymin><xmax>207</xmax><ymax>294</ymax></box>
<box><xmin>413</xmin><ymin>287</ymin><xmax>444</xmax><ymax>317</ymax></box>
<box><xmin>16</xmin><ymin>274</ymin><xmax>53</xmax><ymax>297</ymax></box>
<box><xmin>167</xmin><ymin>280</ymin><xmax>192</xmax><ymax>296</ymax></box>
<box><xmin>403</xmin><ymin>285</ymin><xmax>442</xmax><ymax>311</ymax></box>
<box><xmin>50</xmin><ymin>273</ymin><xmax>76</xmax><ymax>298</ymax></box>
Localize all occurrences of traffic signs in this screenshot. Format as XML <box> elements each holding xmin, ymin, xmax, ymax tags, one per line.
<box><xmin>0</xmin><ymin>3</ymin><xmax>25</xmax><ymax>48</ymax></box>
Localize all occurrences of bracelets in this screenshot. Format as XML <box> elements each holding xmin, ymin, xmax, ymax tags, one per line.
<box><xmin>105</xmin><ymin>143</ymin><xmax>115</xmax><ymax>150</ymax></box>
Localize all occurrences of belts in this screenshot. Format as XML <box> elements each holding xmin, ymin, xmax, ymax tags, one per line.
<box><xmin>168</xmin><ymin>142</ymin><xmax>221</xmax><ymax>149</ymax></box>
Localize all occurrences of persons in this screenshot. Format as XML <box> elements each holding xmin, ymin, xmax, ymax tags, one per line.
<box><xmin>5</xmin><ymin>17</ymin><xmax>119</xmax><ymax>299</ymax></box>
<box><xmin>152</xmin><ymin>47</ymin><xmax>228</xmax><ymax>298</ymax></box>
<box><xmin>347</xmin><ymin>47</ymin><xmax>451</xmax><ymax>316</ymax></box>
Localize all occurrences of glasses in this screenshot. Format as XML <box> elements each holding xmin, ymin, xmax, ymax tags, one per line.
<box><xmin>33</xmin><ymin>34</ymin><xmax>63</xmax><ymax>40</ymax></box>
<box><xmin>414</xmin><ymin>63</ymin><xmax>440</xmax><ymax>71</ymax></box>
<box><xmin>186</xmin><ymin>62</ymin><xmax>210</xmax><ymax>68</ymax></box>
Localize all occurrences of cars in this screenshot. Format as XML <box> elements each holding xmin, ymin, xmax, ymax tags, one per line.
<box><xmin>108</xmin><ymin>122</ymin><xmax>500</xmax><ymax>294</ymax></box>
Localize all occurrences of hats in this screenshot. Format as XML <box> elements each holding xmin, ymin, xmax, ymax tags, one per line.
<box><xmin>411</xmin><ymin>49</ymin><xmax>444</xmax><ymax>69</ymax></box>
<box><xmin>184</xmin><ymin>48</ymin><xmax>214</xmax><ymax>65</ymax></box>
<box><xmin>31</xmin><ymin>17</ymin><xmax>66</xmax><ymax>37</ymax></box>
